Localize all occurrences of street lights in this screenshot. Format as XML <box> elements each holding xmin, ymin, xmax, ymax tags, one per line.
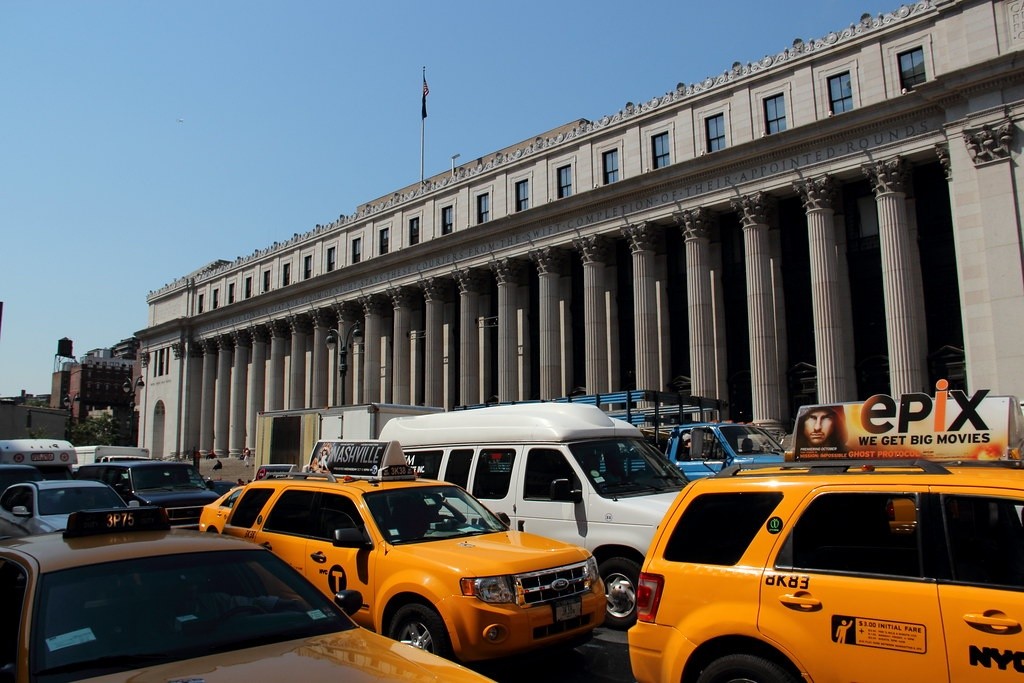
<box><xmin>121</xmin><ymin>375</ymin><xmax>145</xmax><ymax>447</ymax></box>
<box><xmin>63</xmin><ymin>393</ymin><xmax>81</xmax><ymax>441</ymax></box>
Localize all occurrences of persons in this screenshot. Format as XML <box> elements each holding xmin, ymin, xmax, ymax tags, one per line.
<box><xmin>571</xmin><ymin>453</ymin><xmax>623</xmax><ymax>494</ymax></box>
<box><xmin>740</xmin><ymin>437</ymin><xmax>754</xmax><ymax>454</ymax></box>
<box><xmin>133</xmin><ymin>552</ymin><xmax>303</xmax><ymax>651</ymax></box>
<box><xmin>794</xmin><ymin>405</ymin><xmax>849</xmax><ymax>458</ymax></box>
<box><xmin>187</xmin><ymin>445</ymin><xmax>251</xmax><ymax>473</ymax></box>
<box><xmin>424</xmin><ymin>492</ymin><xmax>468</xmax><ymax>529</ymax></box>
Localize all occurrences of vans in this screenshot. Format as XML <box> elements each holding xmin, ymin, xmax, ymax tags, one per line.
<box><xmin>379</xmin><ymin>403</ymin><xmax>691</xmax><ymax>629</ymax></box>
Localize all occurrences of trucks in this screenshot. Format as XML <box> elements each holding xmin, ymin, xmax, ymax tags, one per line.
<box><xmin>453</xmin><ymin>389</ymin><xmax>786</xmax><ymax>482</ymax></box>
<box><xmin>0</xmin><ymin>439</ymin><xmax>78</xmax><ymax>503</ymax></box>
<box><xmin>72</xmin><ymin>445</ymin><xmax>150</xmax><ymax>479</ymax></box>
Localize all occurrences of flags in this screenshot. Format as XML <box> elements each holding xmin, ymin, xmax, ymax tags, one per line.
<box><xmin>421</xmin><ymin>72</ymin><xmax>430</xmax><ymax>119</ymax></box>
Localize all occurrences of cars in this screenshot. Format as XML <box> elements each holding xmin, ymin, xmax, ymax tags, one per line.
<box><xmin>205</xmin><ymin>481</ymin><xmax>242</xmax><ymax>507</ymax></box>
<box><xmin>77</xmin><ymin>461</ymin><xmax>221</xmax><ymax>529</ymax></box>
<box><xmin>628</xmin><ymin>380</ymin><xmax>1024</xmax><ymax>683</ymax></box>
<box><xmin>199</xmin><ymin>440</ymin><xmax>607</xmax><ymax>662</ymax></box>
<box><xmin>0</xmin><ymin>464</ymin><xmax>44</xmax><ymax>497</ymax></box>
<box><xmin>0</xmin><ymin>479</ymin><xmax>129</xmax><ymax>540</ymax></box>
<box><xmin>0</xmin><ymin>507</ymin><xmax>500</xmax><ymax>683</ymax></box>
